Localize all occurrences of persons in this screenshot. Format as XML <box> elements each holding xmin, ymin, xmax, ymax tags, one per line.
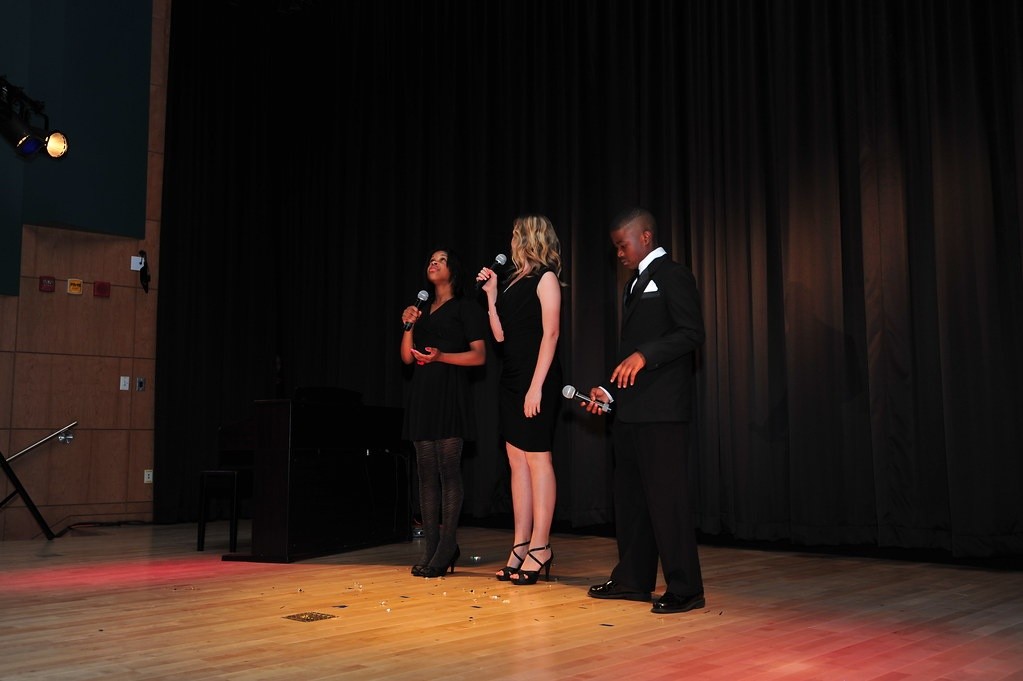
<box><xmin>476</xmin><ymin>213</ymin><xmax>567</xmax><ymax>585</ymax></box>
<box><xmin>579</xmin><ymin>208</ymin><xmax>705</xmax><ymax>614</ymax></box>
<box><xmin>400</xmin><ymin>247</ymin><xmax>486</xmax><ymax>577</ymax></box>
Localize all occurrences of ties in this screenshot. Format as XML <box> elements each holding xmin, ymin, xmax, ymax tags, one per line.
<box><xmin>627</xmin><ymin>268</ymin><xmax>639</xmax><ymax>305</ymax></box>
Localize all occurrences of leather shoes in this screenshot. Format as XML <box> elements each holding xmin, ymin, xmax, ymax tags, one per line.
<box><xmin>650</xmin><ymin>592</ymin><xmax>705</xmax><ymax>613</ymax></box>
<box><xmin>588</xmin><ymin>579</ymin><xmax>651</xmax><ymax>602</ymax></box>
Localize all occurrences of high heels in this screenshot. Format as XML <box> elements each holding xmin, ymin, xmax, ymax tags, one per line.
<box><xmin>495</xmin><ymin>539</ymin><xmax>531</xmax><ymax>581</ymax></box>
<box><xmin>420</xmin><ymin>540</ymin><xmax>461</xmax><ymax>577</ymax></box>
<box><xmin>411</xmin><ymin>537</ymin><xmax>441</xmax><ymax>575</ymax></box>
<box><xmin>510</xmin><ymin>544</ymin><xmax>553</xmax><ymax>584</ymax></box>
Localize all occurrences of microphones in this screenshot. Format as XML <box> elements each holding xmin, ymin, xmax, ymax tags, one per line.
<box><xmin>403</xmin><ymin>290</ymin><xmax>428</xmax><ymax>331</ymax></box>
<box><xmin>562</xmin><ymin>385</ymin><xmax>612</xmax><ymax>414</ymax></box>
<box><xmin>474</xmin><ymin>254</ymin><xmax>507</xmax><ymax>290</ymax></box>
